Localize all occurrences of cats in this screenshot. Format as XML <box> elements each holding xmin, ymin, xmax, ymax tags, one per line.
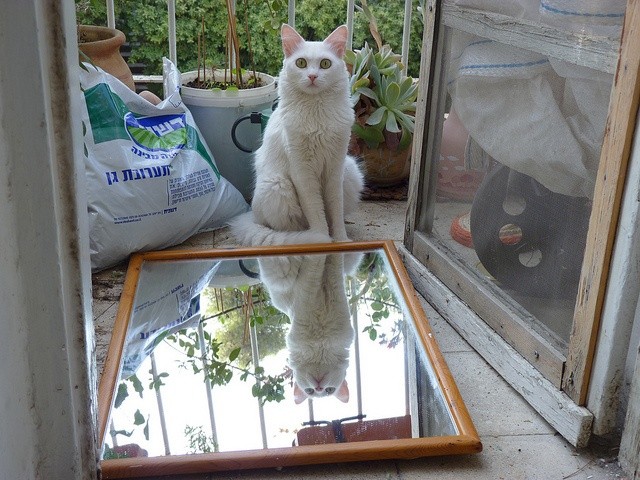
<box><xmin>256</xmin><ymin>252</ymin><xmax>363</xmax><ymax>403</ymax></box>
<box><xmin>228</xmin><ymin>22</ymin><xmax>368</xmax><ymax>244</ymax></box>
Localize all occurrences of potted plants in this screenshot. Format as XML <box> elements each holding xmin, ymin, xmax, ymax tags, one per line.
<box><xmin>355</xmin><ymin>250</ymin><xmax>401</xmax><ymax>343</ymax></box>
<box><xmin>207</xmin><ymin>259</ymin><xmax>282</xmax><ymax>350</ymax></box>
<box><xmin>348</xmin><ymin>40</ymin><xmax>418</xmax><ymax>186</ymax></box>
<box><xmin>177</xmin><ymin>0</ymin><xmax>276</xmax><ymax>203</ymax></box>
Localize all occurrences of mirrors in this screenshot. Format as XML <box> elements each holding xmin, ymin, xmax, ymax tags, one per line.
<box><xmin>100</xmin><ymin>237</ymin><xmax>484</xmax><ymax>471</ymax></box>
